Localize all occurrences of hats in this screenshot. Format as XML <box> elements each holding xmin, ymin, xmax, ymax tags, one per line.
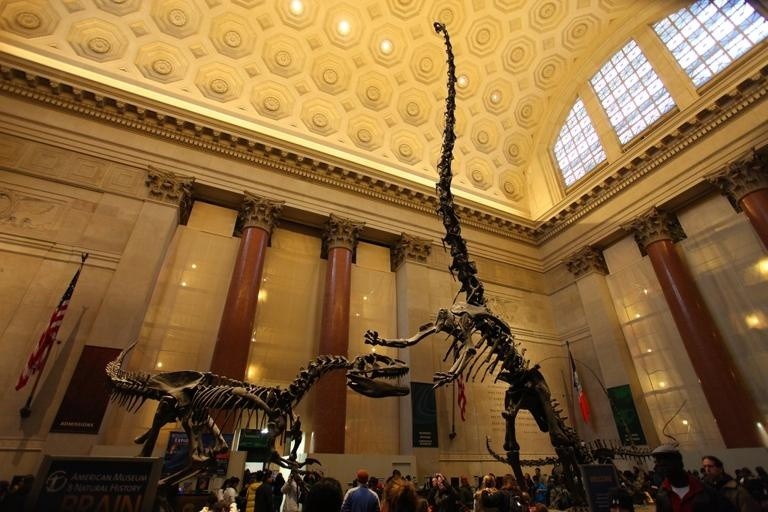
<box><xmin>357</xmin><ymin>469</ymin><xmax>369</xmax><ymax>482</ymax></box>
<box><xmin>651</xmin><ymin>445</ymin><xmax>678</xmax><ymax>458</ymax></box>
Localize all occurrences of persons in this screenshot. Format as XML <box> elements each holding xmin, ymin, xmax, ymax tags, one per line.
<box><xmin>474</xmin><ymin>445</ymin><xmax>767</xmax><ymax>511</ymax></box>
<box><xmin>217</xmin><ymin>468</ymin><xmax>474</xmax><ymax>511</ymax></box>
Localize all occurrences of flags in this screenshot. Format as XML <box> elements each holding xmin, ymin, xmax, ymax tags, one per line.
<box><xmin>454</xmin><ymin>338</ymin><xmax>467</xmax><ymax>422</ymax></box>
<box><xmin>569</xmin><ymin>348</ymin><xmax>591</xmax><ymax>424</ymax></box>
<box><xmin>14</xmin><ymin>262</ymin><xmax>83</xmax><ymax>391</ymax></box>
